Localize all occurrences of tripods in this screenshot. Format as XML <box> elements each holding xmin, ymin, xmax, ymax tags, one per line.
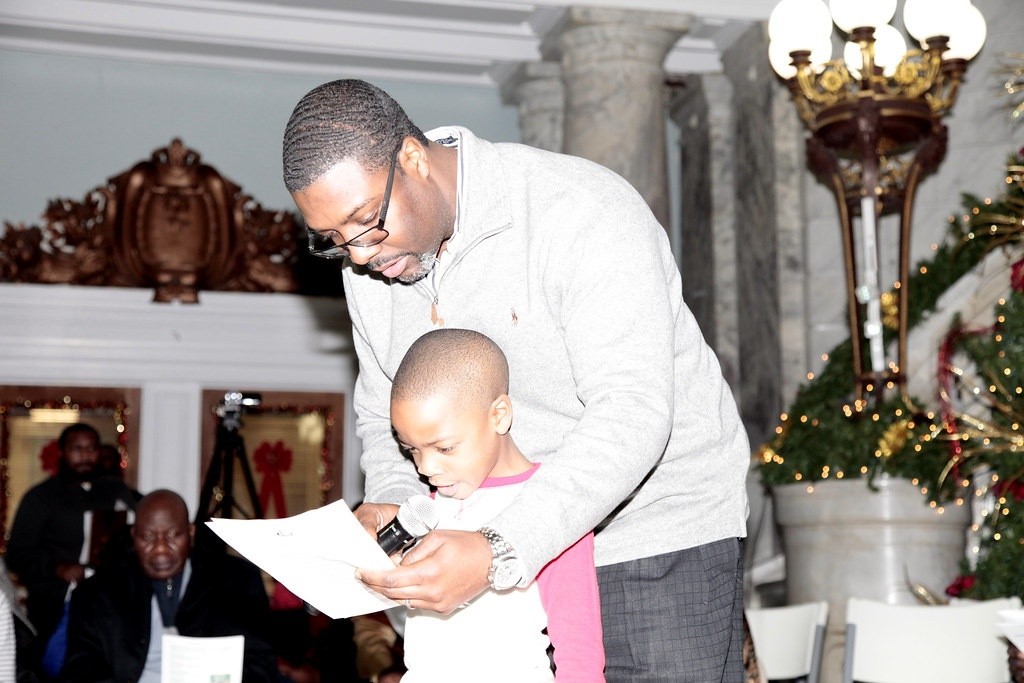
<box><xmin>192</xmin><ymin>419</ymin><xmax>264</xmax><ymax>554</ymax></box>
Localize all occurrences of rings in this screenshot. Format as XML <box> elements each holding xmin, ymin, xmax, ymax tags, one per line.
<box><xmin>403</xmin><ymin>599</ymin><xmax>416</xmax><ymax>615</ymax></box>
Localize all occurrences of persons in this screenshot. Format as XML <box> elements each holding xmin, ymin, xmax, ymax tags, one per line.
<box><xmin>59</xmin><ymin>490</ymin><xmax>282</xmax><ymax>683</ymax></box>
<box><xmin>388</xmin><ymin>329</ymin><xmax>607</xmax><ymax>683</ymax></box>
<box><xmin>284</xmin><ymin>79</ymin><xmax>753</xmax><ymax>683</ymax></box>
<box><xmin>7</xmin><ymin>422</ymin><xmax>145</xmax><ymax>683</ymax></box>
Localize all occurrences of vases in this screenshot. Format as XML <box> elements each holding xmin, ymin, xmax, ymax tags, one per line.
<box><xmin>757</xmin><ymin>394</ymin><xmax>970</xmax><ymax>603</ymax></box>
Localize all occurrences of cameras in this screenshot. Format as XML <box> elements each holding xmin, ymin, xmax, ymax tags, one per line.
<box><xmin>212</xmin><ymin>393</ymin><xmax>261</xmax><ymax>419</ymax></box>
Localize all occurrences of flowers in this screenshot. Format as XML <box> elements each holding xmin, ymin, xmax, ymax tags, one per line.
<box><xmin>945</xmin><ymin>257</ymin><xmax>1024</xmax><ymax>606</ymax></box>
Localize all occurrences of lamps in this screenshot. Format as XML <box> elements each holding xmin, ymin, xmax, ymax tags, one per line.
<box><xmin>767</xmin><ymin>0</ymin><xmax>992</xmax><ymax>405</ymax></box>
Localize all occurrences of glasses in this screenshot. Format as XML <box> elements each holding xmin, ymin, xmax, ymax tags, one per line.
<box><xmin>305</xmin><ymin>141</ymin><xmax>402</xmax><ymax>259</ymax></box>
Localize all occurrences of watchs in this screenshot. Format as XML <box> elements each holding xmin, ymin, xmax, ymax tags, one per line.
<box><xmin>477</xmin><ymin>527</ymin><xmax>523</xmax><ymax>591</ymax></box>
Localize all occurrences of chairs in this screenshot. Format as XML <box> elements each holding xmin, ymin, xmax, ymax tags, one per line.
<box><xmin>745</xmin><ymin>596</ymin><xmax>1024</xmax><ymax>683</ymax></box>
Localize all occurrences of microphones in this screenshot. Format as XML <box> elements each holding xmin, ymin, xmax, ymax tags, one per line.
<box><xmin>304</xmin><ymin>495</ymin><xmax>439</xmax><ymax>616</ymax></box>
